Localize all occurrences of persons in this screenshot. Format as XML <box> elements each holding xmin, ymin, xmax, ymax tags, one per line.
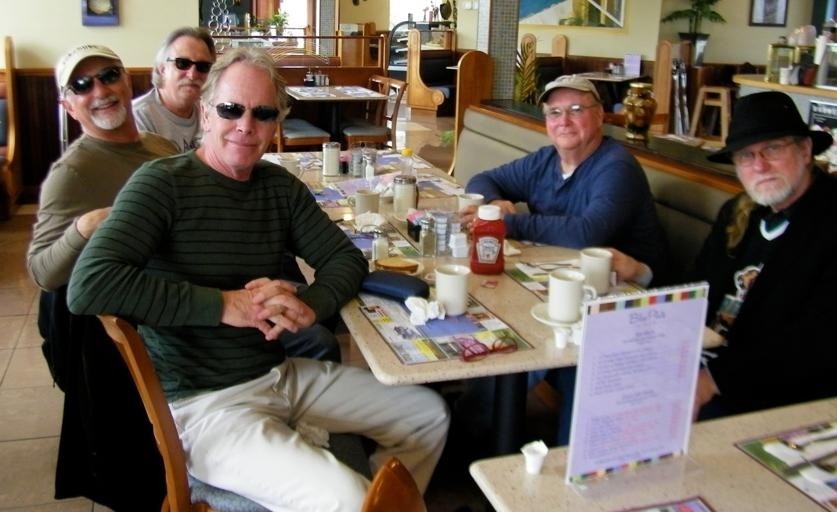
<box><xmin>464</xmin><ymin>74</ymin><xmax>668</xmax><ymax>448</ymax></box>
<box><xmin>26</xmin><ymin>43</ymin><xmax>182</xmax><ymax>292</ymax></box>
<box><xmin>602</xmin><ymin>88</ymin><xmax>837</xmax><ymax>425</ymax></box>
<box><xmin>128</xmin><ymin>26</ymin><xmax>219</xmax><ymax>154</ymax></box>
<box><xmin>68</xmin><ymin>47</ymin><xmax>452</xmax><ymax>512</ymax></box>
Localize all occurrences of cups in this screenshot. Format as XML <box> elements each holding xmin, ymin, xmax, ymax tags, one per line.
<box><xmin>553</xmin><ymin>326</ymin><xmax>572</xmax><ymax>350</ymax></box>
<box><xmin>548</xmin><ymin>268</ymin><xmax>597</xmax><ymax>323</ymax></box>
<box><xmin>520</xmin><ymin>441</ymin><xmax>549</xmax><ymax>474</ymax></box>
<box><xmin>434</xmin><ymin>263</ymin><xmax>472</xmax><ymax>317</ymax></box>
<box><xmin>322</xmin><ymin>142</ymin><xmax>340</xmax><ymax>177</ymax></box>
<box><xmin>778</xmin><ymin>67</ymin><xmax>792</xmax><ymax>86</ymax></box>
<box><xmin>392</xmin><ymin>174</ymin><xmax>420</xmax><ymax>221</ymax></box>
<box><xmin>346</xmin><ymin>189</ymin><xmax>380</xmax><ymax>215</ymax></box>
<box><xmin>458</xmin><ymin>192</ymin><xmax>486</xmax><ymax>209</ymax></box>
<box><xmin>578</xmin><ymin>247</ymin><xmax>613</xmax><ymax>295</ymax></box>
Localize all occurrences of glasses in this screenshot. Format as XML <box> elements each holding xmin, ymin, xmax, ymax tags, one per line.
<box><xmin>463</xmin><ymin>335</ymin><xmax>518</xmax><ymax>361</ymax></box>
<box><xmin>544</xmin><ymin>103</ymin><xmax>598</xmax><ymax>117</ymax></box>
<box><xmin>62</xmin><ymin>66</ymin><xmax>124</xmax><ymax>97</ymax></box>
<box><xmin>208</xmin><ymin>101</ymin><xmax>278</xmax><ymax>121</ymax></box>
<box><xmin>732</xmin><ymin>139</ymin><xmax>800</xmax><ymax>166</ymax></box>
<box><xmin>167</xmin><ymin>57</ymin><xmax>212</xmax><ymax>73</ymax></box>
<box><xmin>339</xmin><ymin>223</ymin><xmax>382</xmax><ymax>240</ymax></box>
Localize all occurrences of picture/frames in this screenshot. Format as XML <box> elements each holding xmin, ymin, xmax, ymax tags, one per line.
<box><xmin>749</xmin><ymin>0</ymin><xmax>788</xmax><ymax>28</ymax></box>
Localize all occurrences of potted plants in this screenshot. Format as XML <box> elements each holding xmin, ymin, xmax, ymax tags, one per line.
<box><xmin>661</xmin><ymin>0</ymin><xmax>728</xmax><ymax>67</ymax></box>
<box><xmin>270</xmin><ymin>9</ymin><xmax>288</xmax><ymax>36</ymax></box>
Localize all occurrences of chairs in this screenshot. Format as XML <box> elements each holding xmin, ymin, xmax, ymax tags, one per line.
<box><xmin>604</xmin><ymin>40</ymin><xmax>673</xmax><ymax>133</ymax></box>
<box><xmin>362</xmin><ymin>457</ymin><xmax>426</xmax><ymax>511</ymax></box>
<box><xmin>269</xmin><ymin>117</ymin><xmax>330</xmax><ymax>152</ymax></box>
<box><xmin>552</xmin><ymin>34</ymin><xmax>567</xmax><ymax>73</ymax></box>
<box><xmin>94</xmin><ymin>314</ymin><xmax>219</xmax><ymax>512</ymax></box>
<box><xmin>408</xmin><ymin>28</ymin><xmax>455</xmax><ymax>116</ymax></box>
<box><xmin>0</xmin><ymin>35</ymin><xmax>23</xmax><ymax>222</ymax></box>
<box><xmin>339</xmin><ymin>74</ymin><xmax>408</xmax><ymax>150</ymax></box>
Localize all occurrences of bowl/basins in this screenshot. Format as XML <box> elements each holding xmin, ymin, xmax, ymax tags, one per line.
<box><xmin>374</xmin><ymin>255</ymin><xmax>424</xmax><ymax>279</ymax></box>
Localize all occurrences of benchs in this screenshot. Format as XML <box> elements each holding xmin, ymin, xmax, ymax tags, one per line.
<box><xmin>454</xmin><ymin>100</ymin><xmax>745</xmax><ymax>275</ymax></box>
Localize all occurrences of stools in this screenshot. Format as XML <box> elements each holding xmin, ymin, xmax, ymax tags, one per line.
<box><xmin>690</xmin><ymin>85</ymin><xmax>733</xmax><ymax>145</ymax></box>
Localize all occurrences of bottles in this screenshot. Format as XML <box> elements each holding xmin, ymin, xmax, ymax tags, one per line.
<box><xmin>370</xmin><ymin>229</ymin><xmax>389</xmax><ymax>261</ymax></box>
<box><xmin>315</xmin><ymin>74</ymin><xmax>329</xmax><ymax>85</ymax></box>
<box><xmin>349</xmin><ymin>148</ymin><xmax>376</xmax><ymax>178</ymax></box>
<box><xmin>399</xmin><ymin>148</ymin><xmax>414</xmax><ymax>176</ymax></box>
<box><xmin>418</xmin><ymin>220</ymin><xmax>436</xmax><ymax>260</ymax></box>
<box><xmin>469</xmin><ymin>205</ymin><xmax>506</xmax><ymax>277</ymax></box>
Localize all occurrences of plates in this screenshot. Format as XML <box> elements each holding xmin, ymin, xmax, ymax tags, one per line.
<box><xmin>531</xmin><ymin>302</ymin><xmax>572</xmax><ymax>327</ymax></box>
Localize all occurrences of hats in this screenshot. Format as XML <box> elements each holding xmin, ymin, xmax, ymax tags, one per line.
<box><xmin>538</xmin><ymin>74</ymin><xmax>600</xmax><ymax>106</ymax></box>
<box><xmin>56</xmin><ymin>44</ymin><xmax>120</xmax><ymax>93</ymax></box>
<box><xmin>706</xmin><ymin>91</ymin><xmax>833</xmax><ymax>163</ymax></box>
